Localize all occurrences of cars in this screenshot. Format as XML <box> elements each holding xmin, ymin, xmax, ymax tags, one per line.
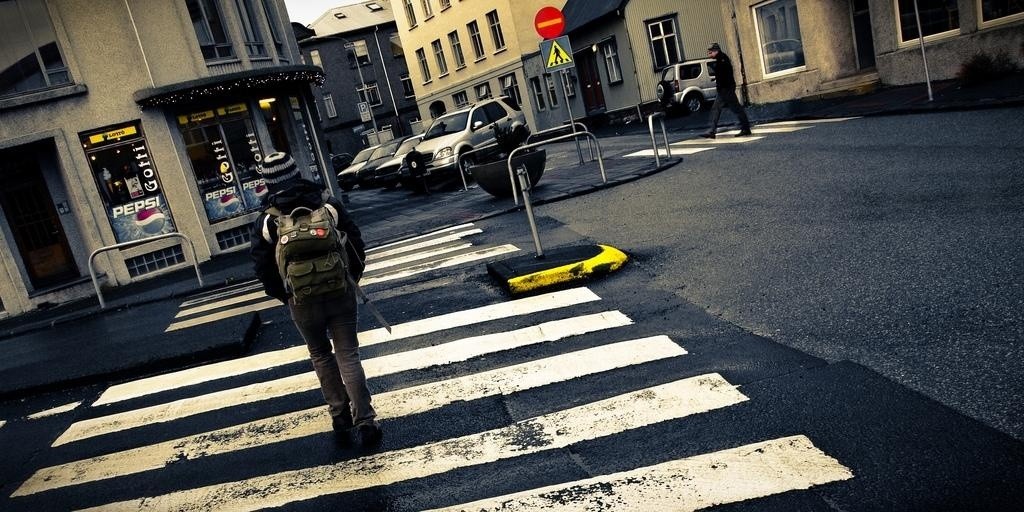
<box><xmin>330</xmin><ymin>131</ymin><xmax>425</xmax><ymax>191</ymax></box>
<box><xmin>764</xmin><ymin>39</ymin><xmax>802</xmax><ymax>72</ymax></box>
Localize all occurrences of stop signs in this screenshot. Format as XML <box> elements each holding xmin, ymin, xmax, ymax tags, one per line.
<box><xmin>534</xmin><ymin>7</ymin><xmax>563</xmax><ymax>37</ymax></box>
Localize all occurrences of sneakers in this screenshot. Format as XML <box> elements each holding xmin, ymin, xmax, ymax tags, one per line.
<box><xmin>735</xmin><ymin>128</ymin><xmax>751</xmax><ymax>137</ymax></box>
<box><xmin>330</xmin><ymin>426</ymin><xmax>383</xmax><ymax>451</ymax></box>
<box><xmin>697</xmin><ymin>132</ymin><xmax>716</xmax><ymax>139</ymax></box>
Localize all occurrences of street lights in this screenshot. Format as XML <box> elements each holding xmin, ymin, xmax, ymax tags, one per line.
<box><xmin>343</xmin><ymin>42</ymin><xmax>382</xmax><ymax>145</ymax></box>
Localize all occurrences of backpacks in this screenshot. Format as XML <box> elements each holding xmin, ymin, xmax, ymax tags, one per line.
<box><xmin>263</xmin><ymin>189</ymin><xmax>350</xmax><ymax>303</ymax></box>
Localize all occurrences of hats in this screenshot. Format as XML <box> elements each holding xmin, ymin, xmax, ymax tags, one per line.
<box><xmin>707</xmin><ymin>42</ymin><xmax>721</xmax><ymax>51</ymax></box>
<box><xmin>261</xmin><ymin>150</ymin><xmax>302</xmax><ymax>191</ymax></box>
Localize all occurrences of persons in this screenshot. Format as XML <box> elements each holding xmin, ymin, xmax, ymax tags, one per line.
<box><xmin>695</xmin><ymin>42</ymin><xmax>752</xmax><ymax>138</ymax></box>
<box><xmin>250</xmin><ymin>149</ymin><xmax>383</xmax><ymax>437</ymax></box>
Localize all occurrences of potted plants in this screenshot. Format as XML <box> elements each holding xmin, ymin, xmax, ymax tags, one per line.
<box><xmin>470</xmin><ymin>120</ymin><xmax>546</xmax><ymax>199</ymax></box>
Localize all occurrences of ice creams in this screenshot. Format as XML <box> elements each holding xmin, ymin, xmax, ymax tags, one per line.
<box><xmin>103</xmin><ymin>168</ymin><xmax>114</xmax><ymax>194</ymax></box>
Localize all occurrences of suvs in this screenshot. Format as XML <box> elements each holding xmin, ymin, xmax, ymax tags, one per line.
<box><xmin>656</xmin><ymin>58</ymin><xmax>716</xmax><ymax>114</ymax></box>
<box><xmin>398</xmin><ymin>94</ymin><xmax>531</xmax><ymax>190</ymax></box>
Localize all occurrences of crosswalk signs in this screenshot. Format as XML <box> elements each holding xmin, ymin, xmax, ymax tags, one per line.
<box><xmin>538</xmin><ymin>34</ymin><xmax>576</xmax><ymax>75</ymax></box>
